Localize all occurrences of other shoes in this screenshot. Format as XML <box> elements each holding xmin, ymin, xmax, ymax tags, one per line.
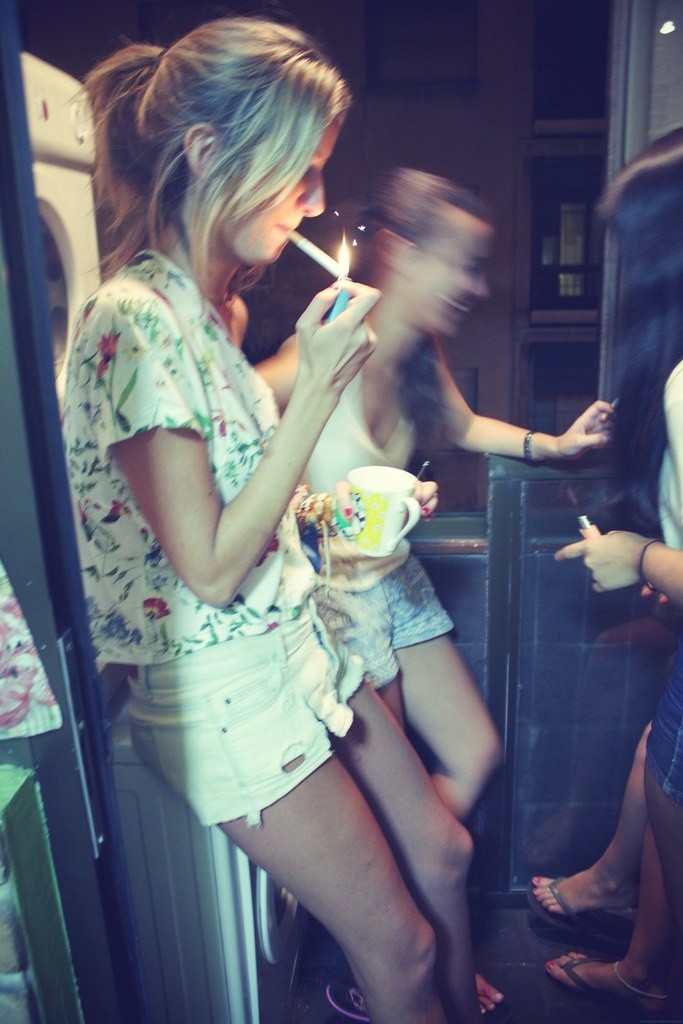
<box><xmin>326</xmin><ymin>980</ymin><xmax>370</xmax><ymax>1022</ymax></box>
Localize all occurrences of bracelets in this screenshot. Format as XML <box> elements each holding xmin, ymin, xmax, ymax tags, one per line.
<box><xmin>639</xmin><ymin>539</ymin><xmax>667</xmax><ymax>591</ymax></box>
<box><xmin>524</xmin><ymin>430</ymin><xmax>540</xmax><ymax>467</ymax></box>
<box><xmin>294</xmin><ymin>484</ymin><xmax>337</xmax><ymax>537</ymax></box>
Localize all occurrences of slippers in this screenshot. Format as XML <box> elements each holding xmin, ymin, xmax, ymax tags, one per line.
<box><xmin>525</xmin><ymin>876</ymin><xmax>635</xmax><ymax>951</ymax></box>
<box><xmin>544</xmin><ymin>952</ymin><xmax>668</xmax><ymax>1022</ymax></box>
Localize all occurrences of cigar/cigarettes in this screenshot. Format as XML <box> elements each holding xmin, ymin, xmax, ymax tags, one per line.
<box><xmin>601</xmin><ymin>397</ymin><xmax>620</xmax><ymax>422</ymax></box>
<box><xmin>288</xmin><ymin>230</ymin><xmax>342</xmax><ymax>279</ymax></box>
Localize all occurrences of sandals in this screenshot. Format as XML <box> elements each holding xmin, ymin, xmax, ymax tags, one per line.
<box><xmin>474</xmin><ymin>971</ymin><xmax>507</xmax><ymax>1024</ymax></box>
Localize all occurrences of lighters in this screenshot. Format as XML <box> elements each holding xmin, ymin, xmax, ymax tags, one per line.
<box><xmin>328</xmin><ymin>276</ymin><xmax>352</xmax><ymax>324</ymax></box>
<box><xmin>577</xmin><ymin>516</ymin><xmax>601</xmax><ymax>540</ymax></box>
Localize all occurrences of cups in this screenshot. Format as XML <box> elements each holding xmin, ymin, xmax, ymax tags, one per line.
<box><xmin>345</xmin><ymin>466</ymin><xmax>421</xmax><ymax>557</ymax></box>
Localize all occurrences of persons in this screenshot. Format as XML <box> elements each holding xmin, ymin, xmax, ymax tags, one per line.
<box><xmin>524</xmin><ymin>126</ymin><xmax>683</xmax><ymax>1024</ymax></box>
<box><xmin>61</xmin><ymin>17</ymin><xmax>473</xmax><ymax>1024</ymax></box>
<box><xmin>249</xmin><ymin>165</ymin><xmax>610</xmax><ymax>1024</ymax></box>
